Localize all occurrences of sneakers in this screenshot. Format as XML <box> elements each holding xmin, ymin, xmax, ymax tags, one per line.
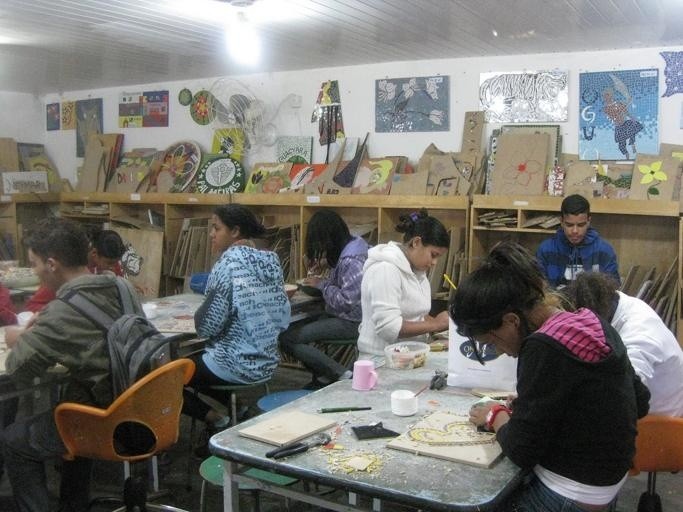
<box><xmin>241</xmin><ymin>404</ymin><xmax>265</xmax><ymax>419</ymax></box>
<box><xmin>191</xmin><ymin>419</ymin><xmax>241</xmax><ymax>463</ymax></box>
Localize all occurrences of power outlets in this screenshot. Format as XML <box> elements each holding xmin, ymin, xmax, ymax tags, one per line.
<box><xmin>290</xmin><ymin>95</ymin><xmax>302</xmax><ymax>109</ymax></box>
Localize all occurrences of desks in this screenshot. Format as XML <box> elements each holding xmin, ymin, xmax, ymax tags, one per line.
<box><xmin>140</xmin><ymin>283</ymin><xmax>323</xmax><ymax>359</ymax></box>
<box><xmin>210</xmin><ymin>331</ymin><xmax>526</xmax><ymax>512</ymax></box>
<box><xmin>0</xmin><ymin>324</ymin><xmax>183</xmax><ymax>404</ymax></box>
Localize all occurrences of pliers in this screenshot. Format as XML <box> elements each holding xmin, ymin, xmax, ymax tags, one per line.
<box><xmin>265</xmin><ymin>432</ymin><xmax>331</xmax><ymax>460</ymax></box>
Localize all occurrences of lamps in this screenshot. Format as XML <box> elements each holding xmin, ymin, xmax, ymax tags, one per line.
<box><xmin>226</xmin><ymin>11</ymin><xmax>260</xmax><ymax>64</ymax></box>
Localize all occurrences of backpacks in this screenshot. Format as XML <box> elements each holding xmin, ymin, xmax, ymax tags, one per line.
<box><xmin>58</xmin><ymin>277</ymin><xmax>178</xmax><ymax>455</ymax></box>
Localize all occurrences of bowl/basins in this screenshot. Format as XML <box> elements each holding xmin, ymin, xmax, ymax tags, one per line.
<box><xmin>384</xmin><ymin>341</ymin><xmax>430</xmax><ymax>370</ymax></box>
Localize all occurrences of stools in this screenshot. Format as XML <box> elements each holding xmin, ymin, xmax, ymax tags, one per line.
<box><xmin>211</xmin><ymin>376</ymin><xmax>275</xmax><ymax>427</ymax></box>
<box><xmin>198</xmin><ymin>456</ymin><xmax>301</xmax><ymax>512</ymax></box>
<box><xmin>257</xmin><ymin>389</ymin><xmax>313</xmax><ymax>413</ymax></box>
<box><xmin>317</xmin><ymin>339</ymin><xmax>360</xmax><ymax>362</ymax></box>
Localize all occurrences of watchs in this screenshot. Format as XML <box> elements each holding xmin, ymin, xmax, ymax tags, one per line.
<box><xmin>486</xmin><ymin>404</ymin><xmax>512</xmax><ymax>430</ymax></box>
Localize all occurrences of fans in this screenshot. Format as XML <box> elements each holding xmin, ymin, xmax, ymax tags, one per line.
<box><xmin>206</xmin><ymin>78</ymin><xmax>278</xmax><ymax>153</ymax></box>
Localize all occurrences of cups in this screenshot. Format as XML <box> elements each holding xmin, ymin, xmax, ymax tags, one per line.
<box><xmin>351</xmin><ymin>360</ymin><xmax>378</xmax><ymax>392</ymax></box>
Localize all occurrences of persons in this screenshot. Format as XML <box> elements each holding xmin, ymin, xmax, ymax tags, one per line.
<box><xmin>358</xmin><ymin>208</ymin><xmax>449</xmax><ymax>369</ymax></box>
<box><xmin>26</xmin><ymin>230</ymin><xmax>126</xmax><ymax>313</ymax></box>
<box><xmin>449</xmin><ymin>241</ymin><xmax>650</xmax><ymax>512</ymax></box>
<box><xmin>558</xmin><ymin>271</ymin><xmax>683</xmax><ymax>417</ymax></box>
<box><xmin>534</xmin><ymin>195</ymin><xmax>620</xmax><ymax>293</ymax></box>
<box><xmin>181</xmin><ymin>204</ymin><xmax>290</xmax><ymax>461</ymax></box>
<box><xmin>0</xmin><ymin>281</ymin><xmax>18</xmax><ymax>327</ymax></box>
<box><xmin>280</xmin><ymin>208</ymin><xmax>372</xmax><ymax>391</ymax></box>
<box><xmin>1</xmin><ymin>217</ymin><xmax>146</xmax><ymax>512</ymax></box>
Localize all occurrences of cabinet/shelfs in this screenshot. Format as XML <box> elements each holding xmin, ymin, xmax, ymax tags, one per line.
<box><xmin>60</xmin><ymin>192</ymin><xmax>230</xmax><ymax>298</ymax></box>
<box><xmin>0</xmin><ymin>194</ymin><xmax>59</xmax><ymax>260</ymax></box>
<box><xmin>231</xmin><ymin>194</ymin><xmax>468</xmax><ymax>317</ymax></box>
<box><xmin>470</xmin><ymin>194</ymin><xmax>683</xmax><ymax>344</ymax></box>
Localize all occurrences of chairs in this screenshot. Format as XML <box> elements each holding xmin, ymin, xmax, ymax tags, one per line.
<box><xmin>54</xmin><ymin>359</ymin><xmax>196</xmax><ymax>511</ymax></box>
<box><xmin>628</xmin><ymin>416</ymin><xmax>683</xmax><ymax>512</ymax></box>
